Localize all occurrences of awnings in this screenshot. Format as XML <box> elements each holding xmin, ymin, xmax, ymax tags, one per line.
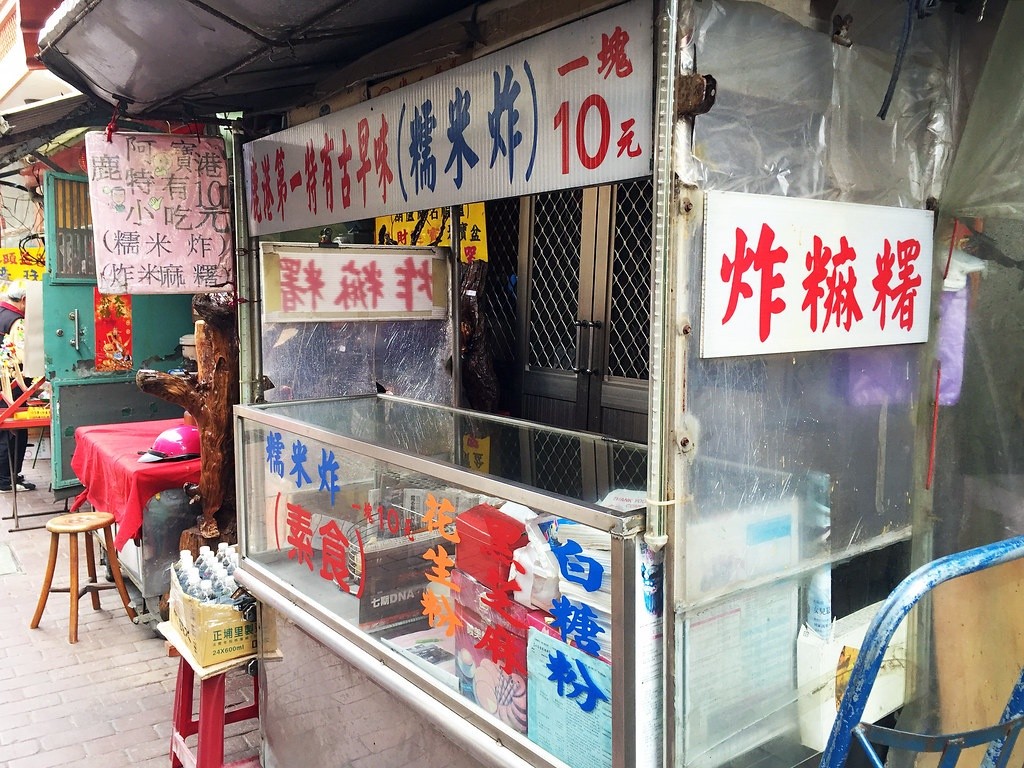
<box><xmin>0</xmin><ymin>93</ymin><xmax>98</xmax><ymax>197</ymax></box>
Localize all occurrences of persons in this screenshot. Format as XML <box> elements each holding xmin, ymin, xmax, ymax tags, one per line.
<box><xmin>829</xmin><ymin>202</ymin><xmax>967</xmax><ymax>595</ymax></box>
<box><xmin>0</xmin><ymin>279</ymin><xmax>37</xmax><ymax>491</ymax></box>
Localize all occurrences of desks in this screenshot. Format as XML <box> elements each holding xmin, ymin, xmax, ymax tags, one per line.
<box><xmin>74</xmin><ymin>419</ymin><xmax>202</xmax><ymax>625</ymax></box>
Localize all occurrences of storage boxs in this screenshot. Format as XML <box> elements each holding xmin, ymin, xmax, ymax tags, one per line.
<box><xmin>169</xmin><ymin>544</ymin><xmax>258</xmax><ymax>668</ymax></box>
<box><xmin>450</xmin><ymin>502</ymin><xmax>613</xmax><ymax>734</ymax></box>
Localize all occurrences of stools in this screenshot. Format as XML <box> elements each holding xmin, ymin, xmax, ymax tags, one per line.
<box><xmin>168</xmin><ymin>656</ymin><xmax>260</xmax><ymax>768</ymax></box>
<box><xmin>30</xmin><ymin>512</ymin><xmax>140</xmax><ymax>643</ymax></box>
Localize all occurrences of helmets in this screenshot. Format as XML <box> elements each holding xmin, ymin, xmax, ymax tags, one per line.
<box><xmin>138</xmin><ymin>423</ymin><xmax>201</xmax><ymax>462</ymax></box>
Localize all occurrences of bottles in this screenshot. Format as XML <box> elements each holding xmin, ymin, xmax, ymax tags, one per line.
<box><xmin>175</xmin><ymin>542</ymin><xmax>239</xmax><ymax>604</ymax></box>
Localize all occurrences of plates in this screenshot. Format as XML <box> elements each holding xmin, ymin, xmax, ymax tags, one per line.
<box><xmin>458</xmin><ymin>650</ymin><xmax>527</xmax><ymax>734</ymax></box>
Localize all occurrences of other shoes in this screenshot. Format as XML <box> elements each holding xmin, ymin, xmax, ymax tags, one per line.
<box><xmin>0</xmin><ymin>482</ymin><xmax>36</xmax><ymax>492</ymax></box>
<box><xmin>17</xmin><ymin>473</ymin><xmax>29</xmax><ymax>483</ymax></box>
